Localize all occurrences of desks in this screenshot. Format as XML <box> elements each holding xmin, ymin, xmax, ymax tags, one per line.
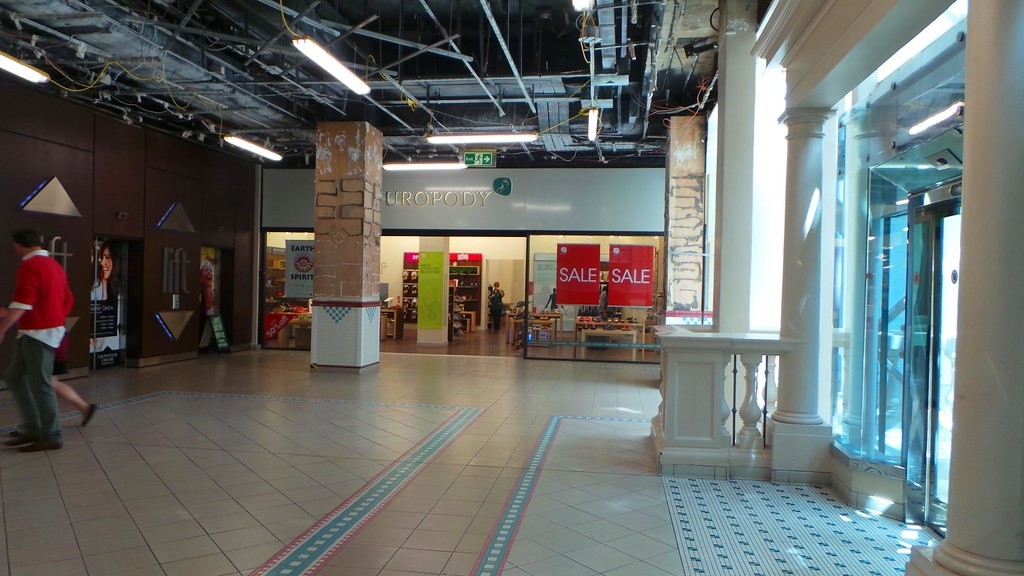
<box><xmin>263</xmin><ymin>312</ymin><xmax>312</xmax><ymax>348</ymax></box>
<box><xmin>460</xmin><ymin>311</ymin><xmax>476</xmax><ymax>332</ymax></box>
<box><xmin>380</xmin><ymin>308</ymin><xmax>404</xmax><ymax>340</ymax></box>
<box><xmin>487</xmin><ymin>303</ymin><xmax>646</xmax><ymax>358</ymax></box>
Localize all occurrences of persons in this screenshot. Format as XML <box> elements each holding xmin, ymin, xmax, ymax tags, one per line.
<box><xmin>0</xmin><ymin>228</ymin><xmax>97</xmax><ymax>452</ymax></box>
<box><xmin>91</xmin><ymin>242</ymin><xmax>122</xmax><ymax>301</ymax></box>
<box><xmin>200</xmin><ymin>259</ymin><xmax>219</xmax><ymax>316</ymax></box>
<box><xmin>488</xmin><ymin>282</ymin><xmax>505</xmax><ymax>333</ymax></box>
<box><xmin>597</xmin><ymin>286</ymin><xmax>609</xmax><ymax>321</ymax></box>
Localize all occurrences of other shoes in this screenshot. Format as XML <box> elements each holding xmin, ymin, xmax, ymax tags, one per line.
<box><xmin>9</xmin><ymin>435</ymin><xmax>36</xmax><ymax>446</ymax></box>
<box><xmin>9</xmin><ymin>431</ymin><xmax>29</xmax><ymax>436</ymax></box>
<box><xmin>81</xmin><ymin>403</ymin><xmax>96</xmax><ymax>427</ymax></box>
<box><xmin>17</xmin><ymin>439</ymin><xmax>63</xmax><ymax>452</ymax></box>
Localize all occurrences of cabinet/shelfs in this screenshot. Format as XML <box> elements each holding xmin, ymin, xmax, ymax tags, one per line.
<box><xmin>403</xmin><ymin>265</ymin><xmax>481</xmax><ymax>323</ymax></box>
<box><xmin>266</xmin><ymin>254</ymin><xmax>309</xmax><ymax>310</ymax></box>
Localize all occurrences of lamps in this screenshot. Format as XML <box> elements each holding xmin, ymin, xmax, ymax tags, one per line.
<box><xmin>587</xmin><ymin>106</ymin><xmax>599</xmax><ymax>142</ymax></box>
<box><xmin>291</xmin><ymin>35</ymin><xmax>371</xmax><ymax>95</ymax></box>
<box><xmin>383</xmin><ymin>161</ymin><xmax>469</xmax><ymax>170</ymax></box>
<box><xmin>224</xmin><ymin>134</ymin><xmax>283</xmax><ymax>162</ymax></box>
<box><xmin>425</xmin><ymin>131</ymin><xmax>539</xmax><ymax>144</ymax></box>
<box><xmin>0</xmin><ymin>50</ymin><xmax>51</xmax><ymax>84</ymax></box>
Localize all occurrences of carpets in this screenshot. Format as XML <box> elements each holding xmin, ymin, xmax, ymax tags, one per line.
<box><xmin>662</xmin><ymin>475</ymin><xmax>939</xmax><ymax>576</ymax></box>
